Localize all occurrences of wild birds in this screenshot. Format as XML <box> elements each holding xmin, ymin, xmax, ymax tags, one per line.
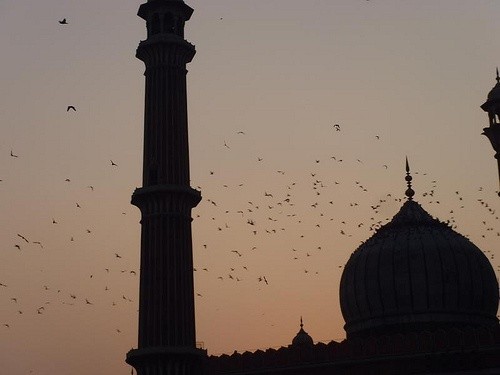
<box><xmin>0</xmin><ymin>176</ymin><xmax>140</xmax><ymax>334</ymax></box>
<box><xmin>191</xmin><ymin>133</ymin><xmax>500</xmax><ymax>286</ymax></box>
<box><xmin>59</xmin><ymin>18</ymin><xmax>68</xmax><ymax>25</ymax></box>
<box><xmin>67</xmin><ymin>105</ymin><xmax>76</xmax><ymax>112</ymax></box>
<box><xmin>333</xmin><ymin>124</ymin><xmax>339</xmax><ymax>127</ymax></box>
<box><xmin>10</xmin><ymin>149</ymin><xmax>19</xmax><ymax>158</ymax></box>
<box><xmin>236</xmin><ymin>131</ymin><xmax>244</xmax><ymax>134</ymax></box>
<box><xmin>110</xmin><ymin>160</ymin><xmax>117</xmax><ymax>167</ymax></box>
<box><xmin>335</xmin><ymin>127</ymin><xmax>340</xmax><ymax>131</ymax></box>
